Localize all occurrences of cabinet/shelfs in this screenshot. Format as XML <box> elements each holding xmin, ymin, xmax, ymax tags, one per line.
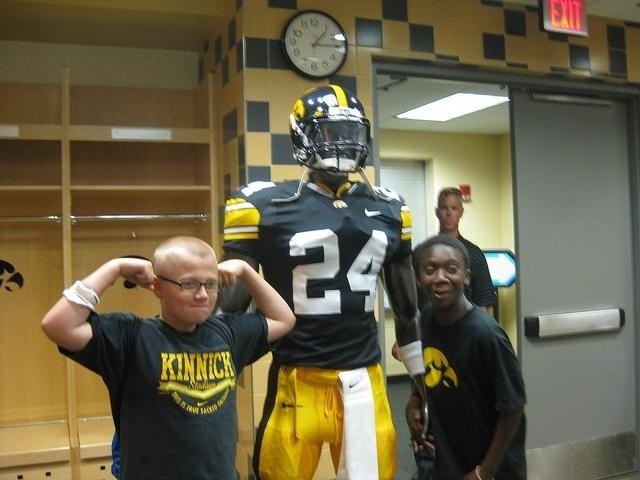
<box><xmin>1</xmin><ymin>69</ymin><xmax>222</xmax><ymax>478</ymax></box>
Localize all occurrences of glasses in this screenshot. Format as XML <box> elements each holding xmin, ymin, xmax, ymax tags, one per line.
<box><xmin>157</xmin><ymin>274</ymin><xmax>223</xmax><ymax>295</ymax></box>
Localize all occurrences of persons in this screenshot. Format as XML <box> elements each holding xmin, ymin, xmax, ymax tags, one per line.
<box><xmin>391</xmin><ymin>186</ymin><xmax>496</xmax><ymax>360</ymax></box>
<box><xmin>552</xmin><ymin>2</ymin><xmax>570</xmax><ymax>32</ymax></box>
<box><xmin>41</xmin><ymin>234</ymin><xmax>296</xmax><ymax>478</ymax></box>
<box><xmin>215</xmin><ymin>81</ymin><xmax>430</xmax><ymax>479</ymax></box>
<box><xmin>403</xmin><ymin>232</ymin><xmax>529</xmax><ymax>480</ymax></box>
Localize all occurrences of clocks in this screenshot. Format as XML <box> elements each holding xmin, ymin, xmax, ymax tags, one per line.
<box><xmin>282</xmin><ymin>9</ymin><xmax>349</xmax><ymax>80</ymax></box>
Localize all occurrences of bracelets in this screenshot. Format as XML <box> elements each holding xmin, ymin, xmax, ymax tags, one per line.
<box><xmin>396</xmin><ymin>337</ymin><xmax>428</xmax><ymax>379</ymax></box>
<box><xmin>62</xmin><ymin>281</ymin><xmax>101</xmax><ymax>312</ymax></box>
<box><xmin>475</xmin><ymin>464</ymin><xmax>496</xmax><ymax>480</ymax></box>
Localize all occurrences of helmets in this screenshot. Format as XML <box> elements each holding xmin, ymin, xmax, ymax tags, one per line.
<box><xmin>288</xmin><ymin>85</ymin><xmax>371</xmax><ymax>174</ymax></box>
